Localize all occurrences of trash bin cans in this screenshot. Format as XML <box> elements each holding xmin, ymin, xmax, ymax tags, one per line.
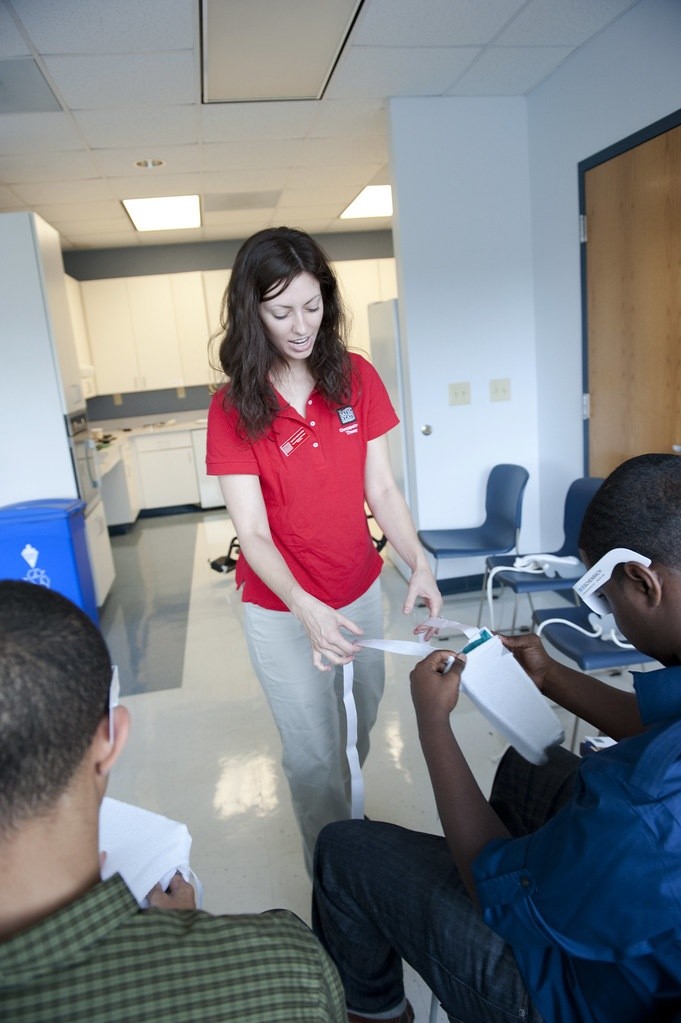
<box><xmin>0</xmin><ymin>497</ymin><xmax>101</xmax><ymax>629</ymax></box>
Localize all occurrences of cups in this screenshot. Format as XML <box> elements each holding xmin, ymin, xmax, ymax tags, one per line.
<box><xmin>92</xmin><ymin>428</ymin><xmax>103</xmax><ymax>441</ymax></box>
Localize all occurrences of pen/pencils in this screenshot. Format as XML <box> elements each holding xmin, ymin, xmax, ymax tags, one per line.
<box><xmin>443</xmin><ymin>656</ymin><xmax>456</xmax><ymax>673</ymax></box>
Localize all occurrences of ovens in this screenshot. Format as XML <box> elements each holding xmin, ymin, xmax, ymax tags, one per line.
<box><xmin>64</xmin><ymin>409</ymin><xmax>102</xmax><ymax>519</ymax></box>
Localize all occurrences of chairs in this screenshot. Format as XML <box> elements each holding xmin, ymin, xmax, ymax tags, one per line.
<box><xmin>531</xmin><ymin>606</ymin><xmax>656</xmax><ymax>755</ymax></box>
<box><xmin>417</xmin><ymin>464</ymin><xmax>530</xmax><ymax>642</ymax></box>
<box><xmin>476</xmin><ymin>476</ymin><xmax>602</xmax><ymax>637</ymax></box>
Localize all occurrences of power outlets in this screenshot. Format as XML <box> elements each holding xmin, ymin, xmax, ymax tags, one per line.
<box><xmin>113</xmin><ymin>394</ymin><xmax>123</xmax><ymax>406</ymax></box>
<box><xmin>176</xmin><ymin>388</ymin><xmax>186</xmax><ymax>399</ymax></box>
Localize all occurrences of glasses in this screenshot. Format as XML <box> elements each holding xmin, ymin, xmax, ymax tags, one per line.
<box><xmin>572</xmin><ymin>547</ymin><xmax>652</xmax><ymax>617</ymax></box>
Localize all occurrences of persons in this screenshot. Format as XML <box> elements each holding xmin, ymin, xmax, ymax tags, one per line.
<box><xmin>204</xmin><ymin>227</ymin><xmax>444</xmax><ymax>891</ymax></box>
<box><xmin>314</xmin><ymin>453</ymin><xmax>681</xmax><ymax>1022</ymax></box>
<box><xmin>0</xmin><ymin>579</ymin><xmax>349</xmax><ymax>1022</ymax></box>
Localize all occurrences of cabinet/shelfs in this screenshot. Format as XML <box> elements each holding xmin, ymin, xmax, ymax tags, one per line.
<box><xmin>170</xmin><ymin>269</ymin><xmax>230</xmax><ymax>387</ymax></box>
<box><xmin>64</xmin><ymin>275</ymin><xmax>97</xmax><ymax>400</ymax></box>
<box><xmin>330</xmin><ymin>257</ymin><xmax>398</xmax><ymax>362</ymax></box>
<box><xmin>77</xmin><ymin>274</ymin><xmax>185</xmax><ymax>396</ymax></box>
<box><xmin>134</xmin><ymin>432</ymin><xmax>200</xmax><ymax>518</ymax></box>
